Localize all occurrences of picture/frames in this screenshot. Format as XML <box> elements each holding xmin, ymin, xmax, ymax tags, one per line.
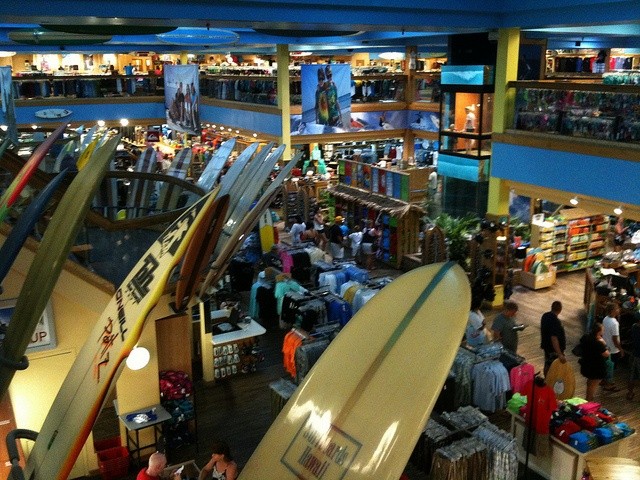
<box><xmin>1</xmin><ymin>295</ymin><xmax>57</xmax><ymax>354</ymax></box>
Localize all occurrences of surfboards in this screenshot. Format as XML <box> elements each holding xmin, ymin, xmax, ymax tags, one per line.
<box><xmin>222</xmin><ymin>142</ymin><xmax>274</xmax><ymax>223</ymax></box>
<box><xmin>4</xmin><ymin>185</ymin><xmax>222</xmax><ymax>480</ymax></box>
<box><xmin>176</xmin><ymin>194</ymin><xmax>230</xmax><ymax>311</ymax></box>
<box><xmin>214</xmin><ymin>142</ymin><xmax>259</xmax><ymax>200</ymax></box>
<box><xmin>210</xmin><ymin>143</ymin><xmax>286</xmax><ymax>265</ymax></box>
<box><xmin>231</xmin><ymin>262</ymin><xmax>473</xmax><ymax>480</ymax></box>
<box><xmin>197</xmin><ymin>138</ymin><xmax>236</xmax><ymax>194</ymax></box>
<box><xmin>256</xmin><ymin>150</ymin><xmax>304</xmax><ymax>199</ymax></box>
<box><xmin>258</xmin><ymin>208</ymin><xmax>274</xmax><ymax>256</ymax></box>
<box><xmin>0</xmin><ymin>135</ymin><xmax>123</xmax><ymax>398</ymax></box>
<box><xmin>155</xmin><ymin>147</ymin><xmax>192</xmax><ymax>231</ymax></box>
<box><xmin>0</xmin><ymin>119</ymin><xmax>71</xmax><ymax>217</ymax></box>
<box><xmin>126</xmin><ymin>147</ymin><xmax>156</xmax><ymax>219</ymax></box>
<box><xmin>88</xmin><ymin>142</ymin><xmax>118</xmax><ymax>221</ymax></box>
<box><xmin>1</xmin><ymin>167</ymin><xmax>69</xmax><ymax>283</ymax></box>
<box><xmin>199</xmin><ymin>177</ymin><xmax>291</xmax><ymax>298</ymax></box>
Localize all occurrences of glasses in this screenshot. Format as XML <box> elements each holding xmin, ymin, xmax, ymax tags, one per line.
<box><xmin>327</xmin><ymin>74</ymin><xmax>331</xmax><ymax>78</ymax></box>
<box><xmin>318</xmin><ymin>77</ymin><xmax>324</xmax><ymax>81</ymax></box>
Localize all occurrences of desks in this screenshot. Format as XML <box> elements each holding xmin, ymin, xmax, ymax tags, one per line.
<box><xmin>585</xmin><ymin>457</ymin><xmax>640</xmax><ymax>480</ymax></box>
<box><xmin>118</xmin><ymin>401</ymin><xmax>174</xmax><ymax>461</ymax></box>
<box><xmin>163</xmin><ymin>458</ymin><xmax>200</xmax><ymax>480</ymax></box>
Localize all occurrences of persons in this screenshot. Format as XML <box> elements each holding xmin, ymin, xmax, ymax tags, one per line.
<box><xmin>622</xmin><ymin>289</ymin><xmax>639</xmax><ymax>412</ymax></box>
<box><xmin>329</xmin><ymin>67</ymin><xmax>344</xmax><ymax>132</ymax></box>
<box><xmin>463</xmin><ymin>107</ymin><xmax>477</xmax><ymax>156</ymax></box>
<box><xmin>613</xmin><ymin>216</ymin><xmax>628</xmax><ymax>252</ymax></box>
<box><xmin>601</xmin><ymin>300</ymin><xmax>622</xmax><ymax>367</ymax></box>
<box><xmin>588</xmin><ymin>282</ymin><xmax>616</xmax><ymax>338</ymax></box>
<box><xmin>574</xmin><ymin>323</ymin><xmax>610</xmax><ymax>404</ymax></box>
<box><xmin>133</xmin><ymin>452</ymin><xmax>183</xmax><ymax>480</ymax></box>
<box><xmin>108</xmin><ymin>65</ymin><xmax>120</xmax><ymax>79</ymax></box>
<box><xmin>315</xmin><ymin>67</ymin><xmax>328</xmax><ymax>132</ymax></box>
<box><xmin>193</xmin><ymin>449</ymin><xmax>241</xmax><ymax>480</ymax></box>
<box><xmin>448</xmin><ymin>124</ymin><xmax>458</xmax><ymax>153</ymax></box>
<box><xmin>465</xmin><ymin>290</ymin><xmax>491</xmax><ymax>354</ymax></box>
<box><xmin>289</xmin><ymin>203</ymin><xmax>385</xmax><ymax>265</ymax></box>
<box><xmin>123</xmin><ymin>63</ymin><xmax>136</xmax><ymax>76</ymax></box>
<box><xmin>493</xmin><ymin>300</ymin><xmax>523</xmax><ymax>370</ymax></box>
<box><xmin>166</xmin><ymin>80</ymin><xmax>198</xmax><ymax>133</ymax></box>
<box><xmin>539</xmin><ymin>299</ymin><xmax>566</xmax><ymax>387</ymax></box>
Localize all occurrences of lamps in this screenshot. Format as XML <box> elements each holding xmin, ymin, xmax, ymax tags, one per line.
<box><xmin>613</xmin><ymin>206</ymin><xmax>626</xmax><ymax>216</ymax></box>
<box><xmin>125</xmin><ymin>344</ymin><xmax>150</xmax><ymax>372</ymax></box>
<box><xmin>569</xmin><ymin>196</ymin><xmax>582</xmax><ymax>206</ymax></box>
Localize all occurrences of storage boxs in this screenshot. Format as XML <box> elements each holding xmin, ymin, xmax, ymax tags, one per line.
<box><xmin>520</xmin><ymin>270</ymin><xmax>552</xmax><ymax>291</ymax></box>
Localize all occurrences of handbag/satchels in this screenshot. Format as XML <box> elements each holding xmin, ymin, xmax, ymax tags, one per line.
<box><xmin>605</xmin><ymin>355</ymin><xmax>614</xmax><ymax>383</ymax></box>
<box><xmin>572</xmin><ymin>344</ymin><xmax>581</xmax><ymax>355</ymax></box>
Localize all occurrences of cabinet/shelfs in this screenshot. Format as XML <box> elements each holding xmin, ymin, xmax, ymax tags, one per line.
<box><xmin>505</xmin><ymin>399</ymin><xmax>636</xmax><ymax>480</ymax></box>
<box><xmin>160</xmin><ymin>388</ymin><xmax>200</xmax><ymax>457</ymax></box>
<box><xmin>582</xmin><ymin>254</ymin><xmax>639</xmax><ymax>335</ymax></box>
<box><xmin>531</xmin><ymin>207</ymin><xmax>611</xmax><ymax>276</ymax></box>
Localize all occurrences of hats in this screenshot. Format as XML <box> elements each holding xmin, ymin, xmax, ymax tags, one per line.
<box><xmin>335</xmin><ymin>216</ymin><xmax>345</xmax><ymax>221</ymax></box>
